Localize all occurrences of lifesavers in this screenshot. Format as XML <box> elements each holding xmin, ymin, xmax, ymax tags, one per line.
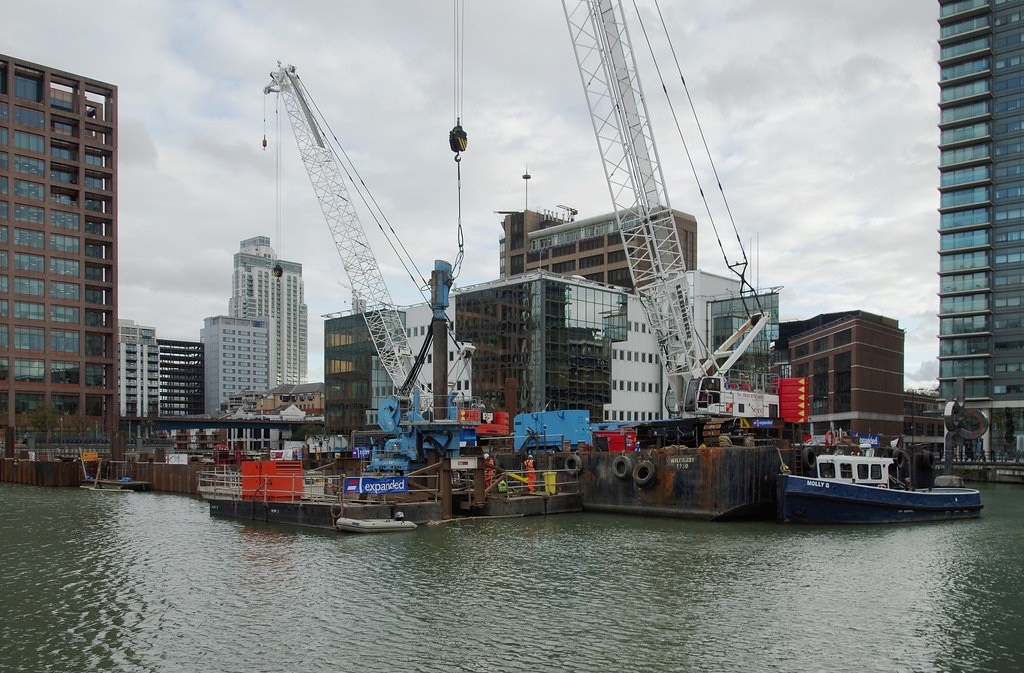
<box><xmin>611</xmin><ymin>455</ymin><xmax>632</xmax><ymax>479</ymax></box>
<box><xmin>803</xmin><ymin>446</ymin><xmax>817</xmax><ymax>470</ymax></box>
<box><xmin>631</xmin><ymin>460</ymin><xmax>656</xmax><ymax>486</ymax></box>
<box><xmin>564</xmin><ymin>454</ymin><xmax>583</xmax><ymax>475</ymax></box>
<box><xmin>824</xmin><ymin>430</ymin><xmax>834</xmax><ymax>446</ymax></box>
<box><xmin>922</xmin><ymin>451</ymin><xmax>936</xmax><ymax>473</ymax></box>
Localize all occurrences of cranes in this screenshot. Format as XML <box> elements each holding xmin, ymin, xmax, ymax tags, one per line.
<box><xmin>263</xmin><ymin>0</ymin><xmax>478</xmax><ymax>471</ymax></box>
<box><xmin>563</xmin><ymin>0</ymin><xmax>769</xmax><ymax>419</ymax></box>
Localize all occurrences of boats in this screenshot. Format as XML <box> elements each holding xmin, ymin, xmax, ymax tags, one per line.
<box><xmin>777</xmin><ymin>444</ymin><xmax>984</xmax><ymax>524</ymax></box>
<box><xmin>80</xmin><ymin>446</ymin><xmax>152</xmax><ymax>492</ymax></box>
<box><xmin>337</xmin><ymin>518</ymin><xmax>417</xmax><ymax>534</ymax></box>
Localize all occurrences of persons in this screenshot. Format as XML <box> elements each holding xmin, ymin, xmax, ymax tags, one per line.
<box><xmin>484</xmin><ymin>454</ymin><xmax>493</xmax><ymax>496</ymax></box>
<box><xmin>524</xmin><ymin>455</ymin><xmax>536</xmax><ymax>492</ymax></box>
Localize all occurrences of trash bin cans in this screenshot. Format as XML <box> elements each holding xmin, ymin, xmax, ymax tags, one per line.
<box><xmin>542</xmin><ymin>472</ymin><xmax>558</xmax><ymax>495</ymax></box>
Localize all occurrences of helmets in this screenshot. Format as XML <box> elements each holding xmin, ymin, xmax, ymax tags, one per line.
<box><xmin>483</xmin><ymin>453</ymin><xmax>489</xmax><ymax>459</ymax></box>
<box><xmin>528</xmin><ymin>455</ymin><xmax>532</xmax><ymax>457</ymax></box>
